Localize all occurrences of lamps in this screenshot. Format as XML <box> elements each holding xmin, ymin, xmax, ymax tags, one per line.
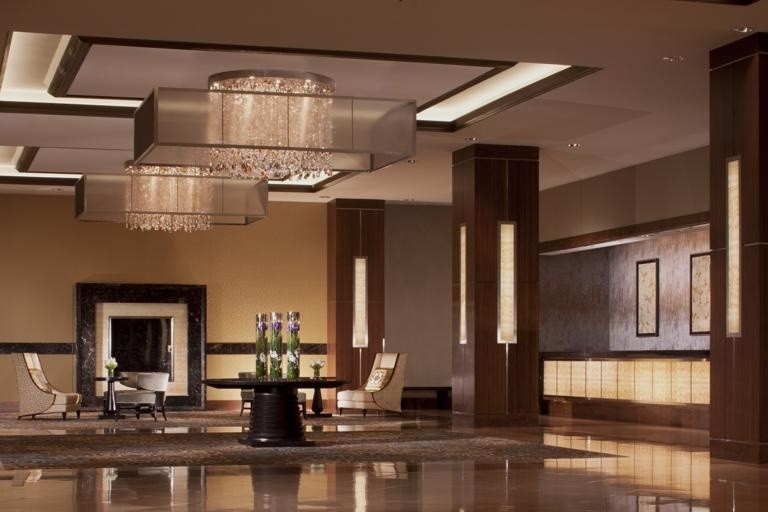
<box><xmin>129</xmin><ymin>71</ymin><xmax>417</xmax><ymax>181</ymax></box>
<box><xmin>74</xmin><ymin>160</ymin><xmax>271</xmax><ymax>235</ymax></box>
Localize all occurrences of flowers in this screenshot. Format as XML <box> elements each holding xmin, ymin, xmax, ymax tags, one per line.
<box><xmin>255</xmin><ymin>312</ymin><xmax>300</xmax><ymax>378</ymax></box>
<box><xmin>106</xmin><ymin>357</ymin><xmax>118</xmax><ymax>369</ymax></box>
<box><xmin>307</xmin><ymin>358</ymin><xmax>326</xmax><ymax>368</ymax></box>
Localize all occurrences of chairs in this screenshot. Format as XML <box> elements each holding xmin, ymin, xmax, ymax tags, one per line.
<box><xmin>104</xmin><ymin>371</ymin><xmax>169</xmax><ymax>422</ymax></box>
<box><xmin>12</xmin><ymin>351</ymin><xmax>82</xmax><ymax>420</ymax></box>
<box><xmin>337</xmin><ymin>352</ymin><xmax>407</xmax><ymax>415</ymax></box>
<box><xmin>239</xmin><ymin>371</ymin><xmax>306</xmax><ymax>416</ymax></box>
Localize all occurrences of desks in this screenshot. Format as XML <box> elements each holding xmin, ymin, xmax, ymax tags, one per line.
<box><xmin>202</xmin><ymin>378</ymin><xmax>348</xmax><ymax>447</ymax></box>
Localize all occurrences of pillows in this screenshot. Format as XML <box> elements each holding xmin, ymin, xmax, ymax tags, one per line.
<box><xmin>27</xmin><ymin>368</ymin><xmax>53</xmax><ymax>394</ymax></box>
<box><xmin>364</xmin><ymin>367</ymin><xmax>393</xmax><ymax>390</ymax></box>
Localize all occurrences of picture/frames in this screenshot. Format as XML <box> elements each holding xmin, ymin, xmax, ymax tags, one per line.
<box><xmin>635</xmin><ymin>258</ymin><xmax>659</xmax><ymax>336</ymax></box>
<box><xmin>689</xmin><ymin>252</ymin><xmax>711</xmax><ymax>335</ymax></box>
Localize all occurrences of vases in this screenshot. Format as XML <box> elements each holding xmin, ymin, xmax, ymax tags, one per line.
<box><xmin>313</xmin><ymin>368</ymin><xmax>320</xmax><ymax>377</ymax></box>
<box><xmin>108</xmin><ymin>368</ymin><xmax>114</xmax><ymax>376</ymax></box>
